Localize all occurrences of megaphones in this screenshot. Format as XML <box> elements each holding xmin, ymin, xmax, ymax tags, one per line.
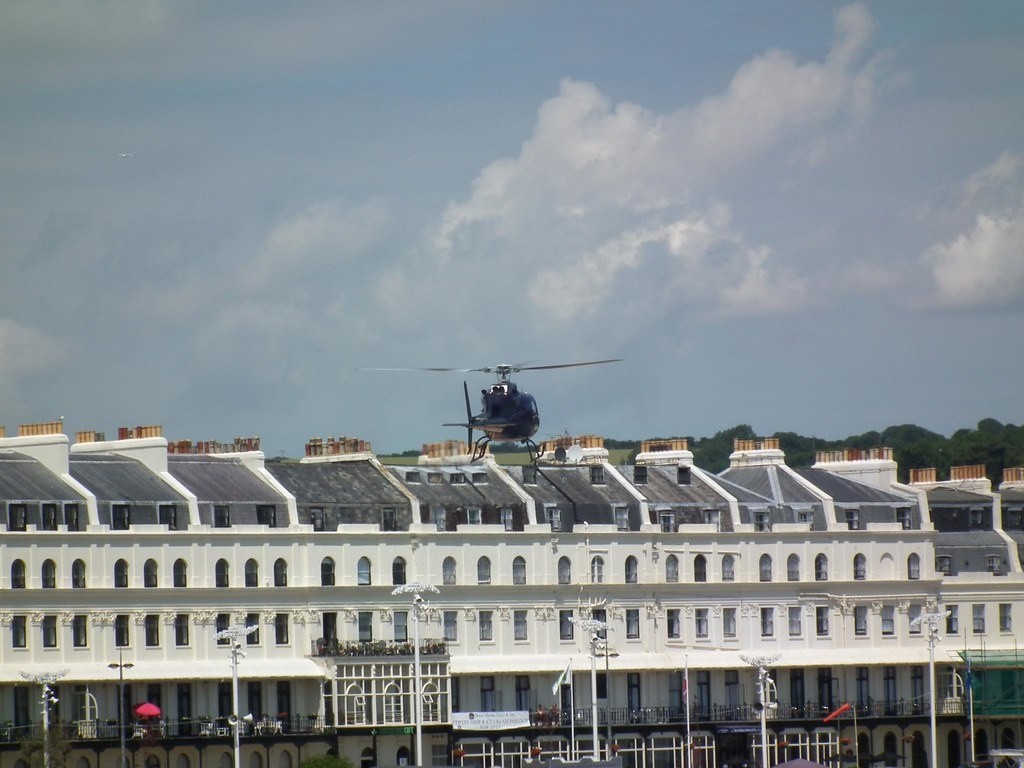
<box><xmin>752</xmin><ymin>702</ymin><xmax>764</xmax><ymax>715</ymax></box>
<box><xmin>242</xmin><ymin>713</ymin><xmax>253</xmax><ymax>725</ymax></box>
<box><xmin>767</xmin><ymin>702</ymin><xmax>778</xmax><ymax>711</ymax></box>
<box><xmin>228</xmin><ymin>714</ymin><xmax>239</xmax><ymax>726</ymax></box>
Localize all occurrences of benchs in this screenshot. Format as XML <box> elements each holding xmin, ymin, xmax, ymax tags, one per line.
<box><xmin>252</xmin><ymin>722</ymin><xmax>282</xmax><ymax>736</ymax></box>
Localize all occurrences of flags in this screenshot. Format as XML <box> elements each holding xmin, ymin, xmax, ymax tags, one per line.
<box><xmin>552</xmin><ymin>664</ymin><xmax>571</xmax><ymax>694</ymax></box>
<box><xmin>965</xmin><ymin>672</ymin><xmax>972</xmax><ymax>716</ymax></box>
<box><xmin>682</xmin><ymin>664</ymin><xmax>687</xmax><ymax>699</ymax></box>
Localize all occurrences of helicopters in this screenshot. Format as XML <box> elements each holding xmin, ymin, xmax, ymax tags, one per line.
<box><xmin>353</xmin><ymin>359</ymin><xmax>624</xmax><ymax>464</ymax></box>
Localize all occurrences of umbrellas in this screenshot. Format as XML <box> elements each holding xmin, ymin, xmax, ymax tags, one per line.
<box><xmin>134</xmin><ymin>703</ymin><xmax>161</xmax><ymax>716</ymax></box>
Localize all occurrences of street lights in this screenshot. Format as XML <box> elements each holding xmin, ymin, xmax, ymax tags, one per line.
<box><xmin>107</xmin><ymin>647</ymin><xmax>134</xmax><ymax>768</ymax></box>
<box><xmin>596</xmin><ymin>628</ymin><xmax>620</xmax><ymax>761</ymax></box>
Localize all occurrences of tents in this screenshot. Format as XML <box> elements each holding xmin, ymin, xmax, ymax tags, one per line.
<box><xmin>772</xmin><ymin>758</ymin><xmax>829</xmax><ymax>768</ymax></box>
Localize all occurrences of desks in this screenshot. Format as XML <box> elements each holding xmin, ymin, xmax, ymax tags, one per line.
<box><xmin>216</xmin><ymin>727</ymin><xmax>229</xmax><ymax>736</ymax></box>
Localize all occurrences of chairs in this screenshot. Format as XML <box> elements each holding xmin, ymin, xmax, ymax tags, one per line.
<box><xmin>230</xmin><ymin>720</ymin><xmax>246</xmax><ymax>736</ymax></box>
<box><xmin>199</xmin><ymin>723</ymin><xmax>214</xmax><ymax>737</ymax></box>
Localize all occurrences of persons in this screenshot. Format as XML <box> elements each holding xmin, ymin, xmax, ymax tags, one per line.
<box><xmin>137</xmin><ymin>715</ymin><xmax>166</xmax><ymax>735</ymax></box>
<box><xmin>1</xmin><ymin>720</ymin><xmax>17</xmax><ymax>741</ymax></box>
<box><xmin>535</xmin><ymin>703</ymin><xmax>558</xmax><ymax>726</ymax></box>
